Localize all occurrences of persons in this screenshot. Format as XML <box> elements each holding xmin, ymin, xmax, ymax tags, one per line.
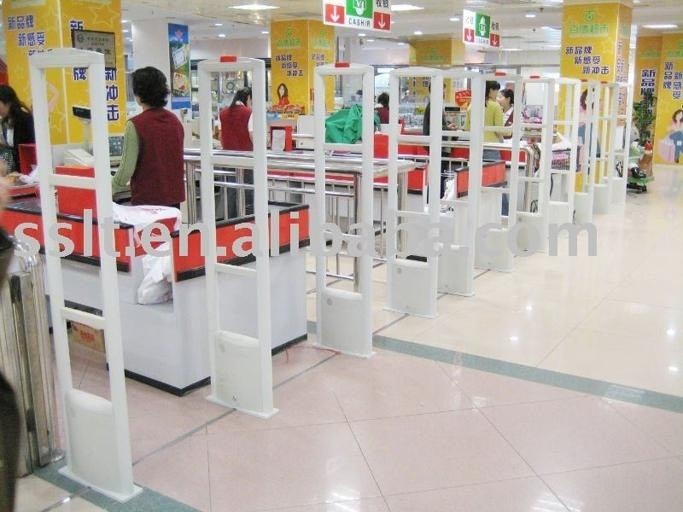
<box><xmin>580</xmin><ymin>88</ymin><xmax>594</xmax><ymax>112</ymax></box>
<box><xmin>375</xmin><ymin>92</ymin><xmax>389</xmax><ymax>123</ymax></box>
<box><xmin>217</xmin><ymin>86</ymin><xmax>253</xmax><ymax>151</ymax></box>
<box><xmin>497</xmin><ymin>89</ymin><xmax>514</xmax><ymax>138</ymax></box>
<box><xmin>112</xmin><ymin>64</ymin><xmax>186</xmax><ymax>210</ymax></box>
<box><xmin>463</xmin><ymin>81</ymin><xmax>504</xmax><ymax>160</ymax></box>
<box><xmin>0</xmin><ymin>82</ymin><xmax>35</xmax><ymax>173</ymax></box>
<box><xmin>277</xmin><ymin>83</ymin><xmax>289</xmax><ymax>105</ymax></box>
<box><xmin>423</xmin><ymin>81</ymin><xmax>458</xmax><ymax>202</ymax></box>
<box><xmin>0</xmin><ymin>225</ymin><xmax>21</xmax><ymax>511</ymax></box>
<box><xmin>658</xmin><ymin>109</ymin><xmax>683</xmax><ymax>163</ymax></box>
